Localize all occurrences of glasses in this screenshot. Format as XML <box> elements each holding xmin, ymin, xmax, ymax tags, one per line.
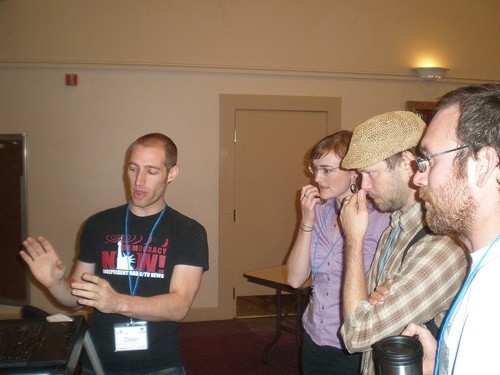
<box><xmin>309</xmin><ymin>166</ymin><xmax>340</xmax><ymax>175</ymax></box>
<box><xmin>416</xmin><ymin>145</ymin><xmax>469</xmax><ymax>173</ymax></box>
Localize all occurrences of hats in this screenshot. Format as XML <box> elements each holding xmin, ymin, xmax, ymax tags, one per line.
<box><xmin>341</xmin><ymin>111</ymin><xmax>427</xmax><ymax>169</ymax></box>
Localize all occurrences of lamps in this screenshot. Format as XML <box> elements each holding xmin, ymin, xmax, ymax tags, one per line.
<box><xmin>412</xmin><ymin>67</ymin><xmax>450</xmax><ymax>81</ymax></box>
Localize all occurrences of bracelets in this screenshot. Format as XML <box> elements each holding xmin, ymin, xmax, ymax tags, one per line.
<box><xmin>301</xmin><ymin>226</ymin><xmax>313</xmax><ymax>232</ymax></box>
<box><xmin>300</xmin><ymin>222</ymin><xmax>312</xmax><ymax>228</ymax></box>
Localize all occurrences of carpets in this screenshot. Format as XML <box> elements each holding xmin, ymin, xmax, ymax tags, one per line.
<box><xmin>180</xmin><ymin>331</ymin><xmax>303</xmax><ymax>375</ymax></box>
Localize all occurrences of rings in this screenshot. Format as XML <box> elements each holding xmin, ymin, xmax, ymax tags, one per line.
<box><xmin>343</xmin><ymin>199</ymin><xmax>350</xmax><ymax>202</ymax></box>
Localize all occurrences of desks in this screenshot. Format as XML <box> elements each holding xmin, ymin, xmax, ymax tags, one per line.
<box><xmin>0</xmin><ymin>315</ymin><xmax>105</xmax><ymax>375</ymax></box>
<box><xmin>243</xmin><ymin>266</ymin><xmax>312</xmax><ymax>375</ymax></box>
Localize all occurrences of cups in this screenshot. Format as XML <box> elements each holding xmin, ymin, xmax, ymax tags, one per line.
<box><xmin>371</xmin><ymin>335</ymin><xmax>423</xmax><ymax>375</ymax></box>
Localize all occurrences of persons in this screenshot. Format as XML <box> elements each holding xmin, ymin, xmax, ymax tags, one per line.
<box><xmin>412</xmin><ymin>83</ymin><xmax>500</xmax><ymax>375</ymax></box>
<box><xmin>20</xmin><ymin>133</ymin><xmax>209</xmax><ymax>375</ymax></box>
<box><xmin>287</xmin><ymin>130</ymin><xmax>392</xmax><ymax>375</ymax></box>
<box><xmin>340</xmin><ymin>111</ymin><xmax>472</xmax><ymax>375</ymax></box>
